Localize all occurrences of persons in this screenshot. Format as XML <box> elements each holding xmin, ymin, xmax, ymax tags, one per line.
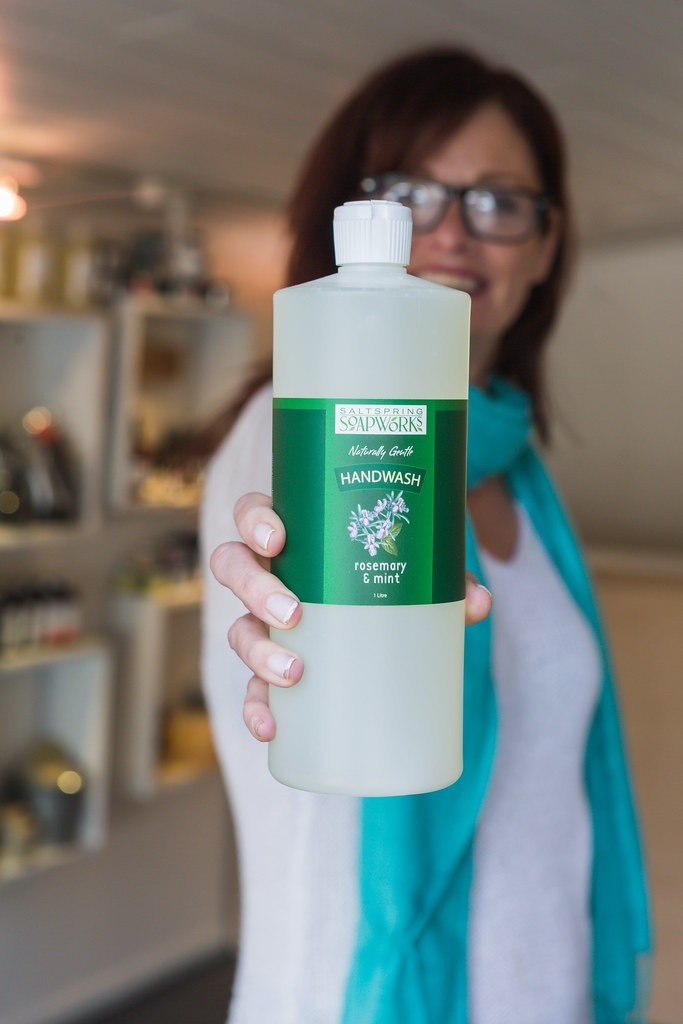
<box><xmin>198</xmin><ymin>45</ymin><xmax>654</xmax><ymax>1024</ymax></box>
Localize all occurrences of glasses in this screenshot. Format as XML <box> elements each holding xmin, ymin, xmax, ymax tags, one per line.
<box><xmin>357</xmin><ymin>171</ymin><xmax>555</xmax><ymax>246</ymax></box>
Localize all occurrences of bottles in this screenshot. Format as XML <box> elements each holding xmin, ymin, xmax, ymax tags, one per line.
<box><xmin>265</xmin><ymin>200</ymin><xmax>471</xmax><ymax>797</ymax></box>
